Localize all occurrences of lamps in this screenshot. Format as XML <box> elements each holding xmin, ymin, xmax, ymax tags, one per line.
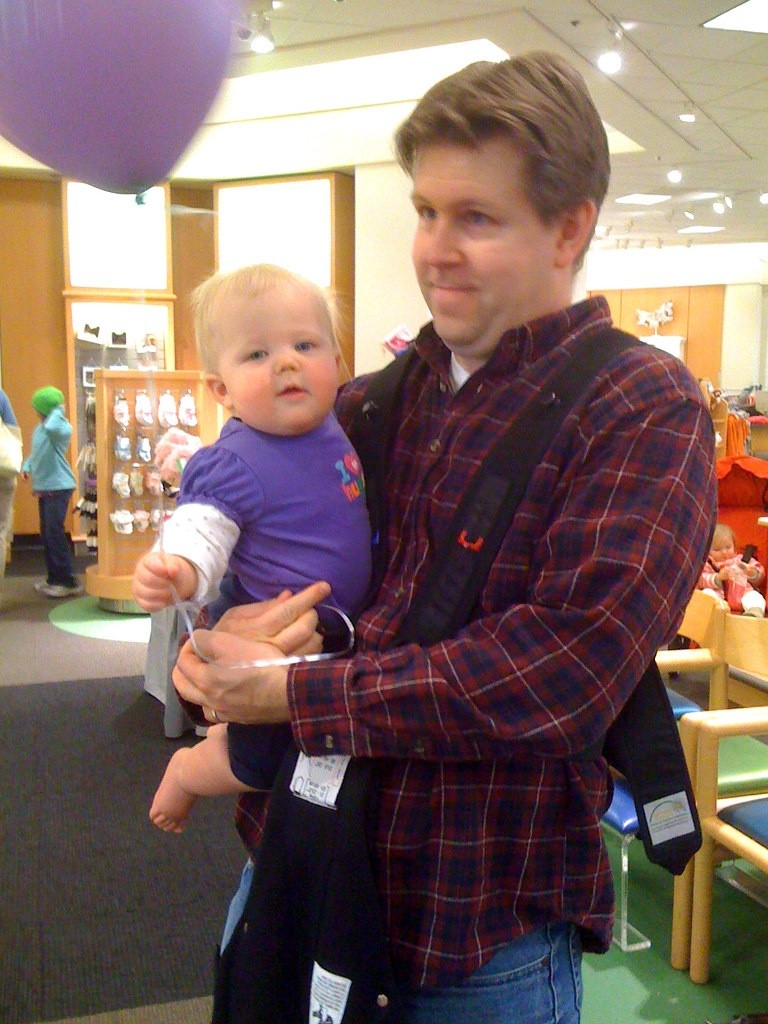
<box><xmin>598</xmin><ymin>32</ymin><xmax>622</xmax><ymax>73</ymax></box>
<box><xmin>759</xmin><ymin>190</ymin><xmax>768</xmax><ymax>204</ymax></box>
<box><xmin>679</xmin><ymin>100</ymin><xmax>696</xmax><ymax>122</ymax></box>
<box><xmin>725</xmin><ymin>196</ymin><xmax>736</xmax><ymax>208</ymax></box>
<box><xmin>600</xmin><ymin>205</ymin><xmax>697</xmax><ymax>251</ymax></box>
<box><xmin>251</xmin><ymin>14</ymin><xmax>274</xmax><ymax>54</ymax></box>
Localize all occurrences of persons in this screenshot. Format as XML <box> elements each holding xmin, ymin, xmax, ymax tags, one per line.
<box><xmin>131</xmin><ymin>259</ymin><xmax>373</xmax><ymax>835</ymax></box>
<box><xmin>0</xmin><ymin>385</ymin><xmax>24</xmax><ymax>578</ymax></box>
<box><xmin>22</xmin><ymin>386</ymin><xmax>84</xmax><ymax>597</ymax></box>
<box><xmin>695</xmin><ymin>523</ymin><xmax>765</xmax><ymax>617</ymax></box>
<box><xmin>172</xmin><ymin>52</ymin><xmax>714</xmax><ymax>1024</ymax></box>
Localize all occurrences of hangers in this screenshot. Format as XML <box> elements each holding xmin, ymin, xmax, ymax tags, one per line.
<box><xmin>727</xmin><ymin>395</ymin><xmax>739</xmax><ymax>415</ymax></box>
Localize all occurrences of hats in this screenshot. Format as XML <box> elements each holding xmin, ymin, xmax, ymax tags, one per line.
<box><xmin>32</xmin><ymin>386</ymin><xmax>65</xmax><ymax>416</ymax></box>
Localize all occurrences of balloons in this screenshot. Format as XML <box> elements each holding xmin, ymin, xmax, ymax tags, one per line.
<box><xmin>0</xmin><ymin>0</ymin><xmax>246</xmax><ymax>205</ymax></box>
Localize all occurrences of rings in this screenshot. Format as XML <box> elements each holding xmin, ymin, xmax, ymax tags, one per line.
<box><xmin>212</xmin><ymin>708</ymin><xmax>225</xmax><ymax>723</ymax></box>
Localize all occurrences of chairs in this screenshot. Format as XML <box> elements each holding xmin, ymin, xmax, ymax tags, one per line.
<box><xmin>604</xmin><ymin>589</ymin><xmax>768</xmax><ymax>985</ymax></box>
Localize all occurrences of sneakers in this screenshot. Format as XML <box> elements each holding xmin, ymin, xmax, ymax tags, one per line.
<box><xmin>35</xmin><ymin>579</ymin><xmax>85</xmax><ymax>597</ymax></box>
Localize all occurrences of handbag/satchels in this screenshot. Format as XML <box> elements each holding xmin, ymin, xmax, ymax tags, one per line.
<box><xmin>0</xmin><ymin>415</ymin><xmax>23</xmax><ymax>479</ymax></box>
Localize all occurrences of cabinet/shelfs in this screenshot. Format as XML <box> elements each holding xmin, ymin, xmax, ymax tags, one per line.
<box><xmin>710</xmin><ymin>399</ymin><xmax>729</xmax><ymax>461</ymax></box>
<box><xmin>745</xmin><ymin>420</ymin><xmax>768</xmax><ymax>457</ymax></box>
